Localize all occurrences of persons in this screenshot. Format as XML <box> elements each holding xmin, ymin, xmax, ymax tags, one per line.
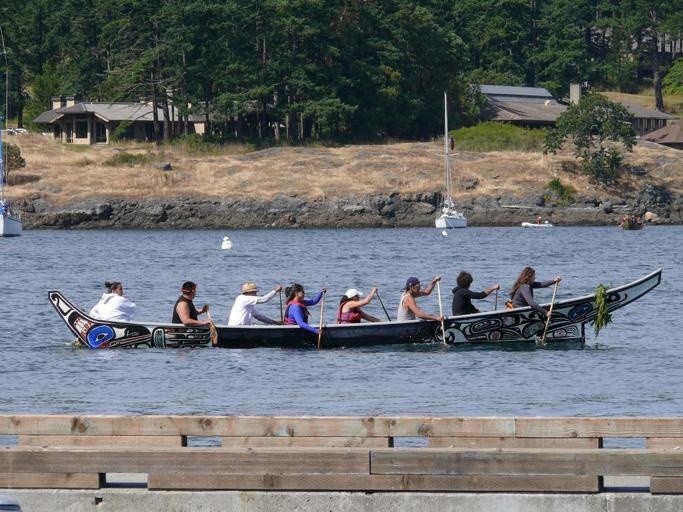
<box><xmin>509</xmin><ymin>267</ymin><xmax>561</xmax><ymax>317</ymax></box>
<box><xmin>535</xmin><ymin>215</ymin><xmax>542</xmax><ymax>224</ymax></box>
<box><xmin>452</xmin><ymin>271</ymin><xmax>500</xmax><ymax>315</ymax></box>
<box><xmin>171</xmin><ymin>281</ymin><xmax>213</xmax><ymax>326</ymax></box>
<box><xmin>337</xmin><ymin>288</ymin><xmax>385</xmax><ymax>324</ymax></box>
<box><xmin>282</xmin><ymin>285</ymin><xmax>327</xmax><ymax>334</ymax></box>
<box><xmin>89</xmin><ymin>281</ymin><xmax>137</xmax><ymax>322</ymax></box>
<box><xmin>397</xmin><ymin>276</ymin><xmax>445</xmax><ymax>321</ymax></box>
<box><xmin>228</xmin><ymin>283</ymin><xmax>283</xmax><ymax>326</ymax></box>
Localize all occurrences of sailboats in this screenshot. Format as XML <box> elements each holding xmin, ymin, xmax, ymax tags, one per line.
<box><xmin>434</xmin><ymin>92</ymin><xmax>472</xmax><ymax>229</ymax></box>
<box><xmin>0</xmin><ymin>27</ymin><xmax>21</xmax><ymax>237</ymax></box>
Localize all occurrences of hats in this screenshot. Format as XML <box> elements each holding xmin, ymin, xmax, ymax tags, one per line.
<box><xmin>343</xmin><ymin>288</ymin><xmax>364</xmax><ymax>299</ymax></box>
<box><xmin>240</xmin><ymin>282</ymin><xmax>260</xmax><ymax>293</ymax></box>
<box><xmin>402</xmin><ymin>276</ymin><xmax>420</xmax><ymax>290</ymax></box>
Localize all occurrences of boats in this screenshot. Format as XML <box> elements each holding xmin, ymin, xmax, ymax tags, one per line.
<box><xmin>521</xmin><ymin>221</ymin><xmax>553</xmax><ymax>229</ymax></box>
<box><xmin>46</xmin><ymin>268</ymin><xmax>664</xmax><ymax>352</ymax></box>
<box><xmin>622</xmin><ymin>217</ymin><xmax>644</xmax><ymax>231</ymax></box>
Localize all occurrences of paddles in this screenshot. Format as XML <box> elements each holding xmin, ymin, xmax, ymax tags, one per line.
<box><xmin>539</xmin><ymin>279</ymin><xmax>558</xmax><ymax>349</ymax></box>
<box><xmin>206</xmin><ymin>305</ymin><xmax>218</xmax><ymax>344</ymax></box>
<box><xmin>437</xmin><ymin>279</ymin><xmax>449</xmax><ymax>348</ymax></box>
<box><xmin>317</xmin><ymin>291</ymin><xmax>325</xmax><ymax>350</ymax></box>
